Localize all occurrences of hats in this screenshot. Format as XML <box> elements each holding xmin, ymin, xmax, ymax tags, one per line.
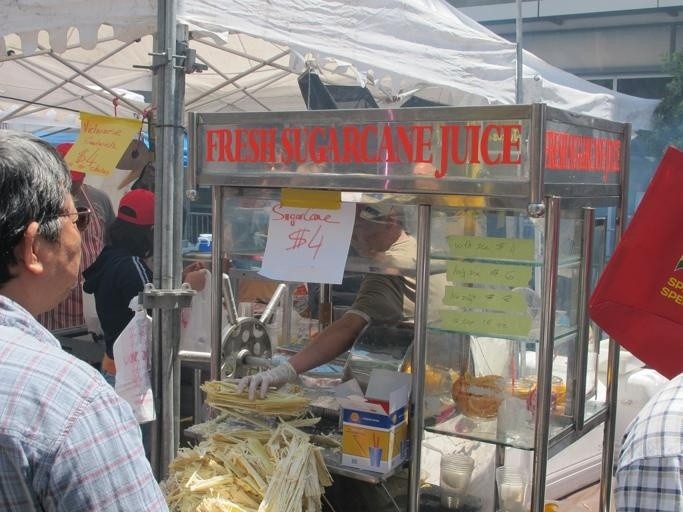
<box><xmin>359</xmin><ymin>204</ymin><xmax>405</xmax><ymax>226</ymax></box>
<box><xmin>56</xmin><ymin>143</ymin><xmax>84</xmax><ymax>182</ymax></box>
<box><xmin>118</xmin><ymin>189</ymin><xmax>154</xmax><ymax>225</ymax></box>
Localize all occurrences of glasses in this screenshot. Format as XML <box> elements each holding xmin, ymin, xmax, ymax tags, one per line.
<box><xmin>25</xmin><ymin>206</ymin><xmax>93</xmax><ymax>232</ymax></box>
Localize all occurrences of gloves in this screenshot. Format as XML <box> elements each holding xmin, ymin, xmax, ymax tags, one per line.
<box><xmin>237</xmin><ymin>359</ymin><xmax>298</xmax><ymax>401</ymax></box>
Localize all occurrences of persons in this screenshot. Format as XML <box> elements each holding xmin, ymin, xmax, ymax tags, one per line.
<box><xmin>0</xmin><ymin>127</ymin><xmax>169</xmax><ymax>511</ymax></box>
<box><xmin>246</xmin><ymin>138</ymin><xmax>290</xmax><ymax>198</ymax></box>
<box><xmin>224</xmin><ymin>198</ymin><xmax>468</xmax><ymax>402</ymax></box>
<box><xmin>81</xmin><ymin>188</ymin><xmax>209</xmax><ymax>389</ymax></box>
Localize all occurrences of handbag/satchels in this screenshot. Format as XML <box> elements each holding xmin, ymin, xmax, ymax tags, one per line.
<box><xmin>588</xmin><ymin>145</ymin><xmax>683</xmax><ymax>380</ymax></box>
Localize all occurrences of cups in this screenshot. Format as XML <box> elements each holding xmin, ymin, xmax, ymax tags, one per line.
<box><xmin>369</xmin><ymin>446</ymin><xmax>383</xmax><ymax>467</ymax></box>
<box><xmin>495</xmin><ymin>465</ymin><xmax>529</xmax><ymax>512</ymax></box>
<box><xmin>439</xmin><ymin>454</ymin><xmax>475</xmax><ymax>512</ymax></box>
<box><xmin>494</xmin><ymin>374</ymin><xmax>564</xmax><ymax>448</ymax></box>
